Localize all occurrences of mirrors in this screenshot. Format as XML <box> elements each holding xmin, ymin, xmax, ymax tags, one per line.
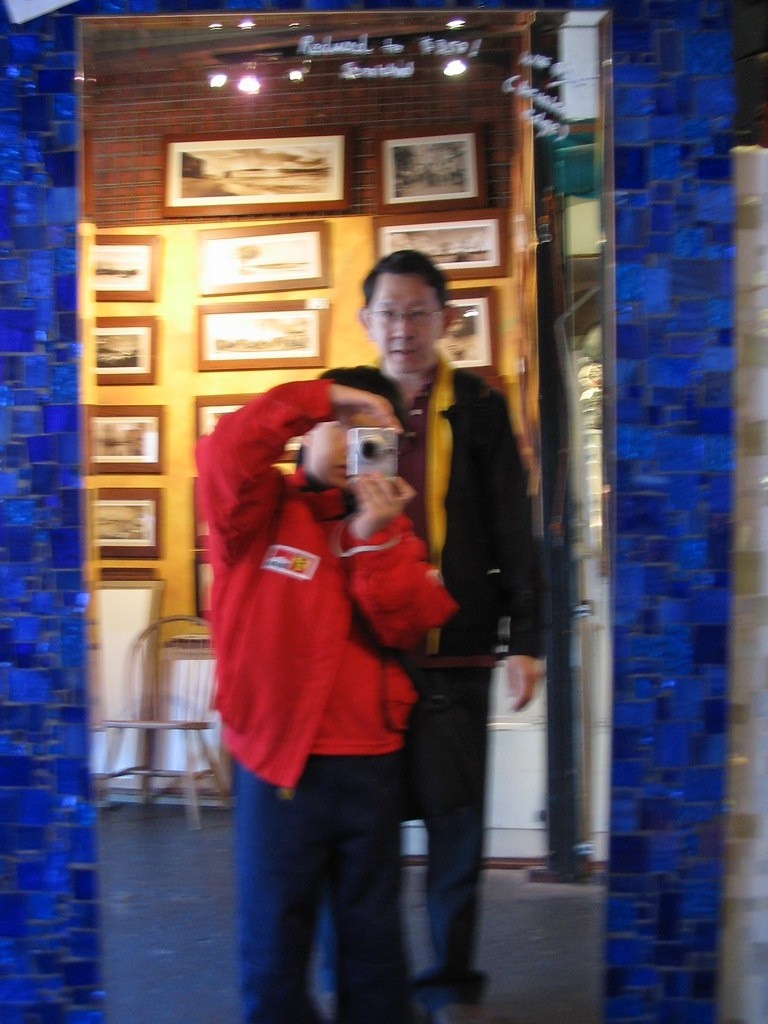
<box><xmin>78</xmin><ymin>8</ymin><xmax>609</xmax><ymax>1024</ymax></box>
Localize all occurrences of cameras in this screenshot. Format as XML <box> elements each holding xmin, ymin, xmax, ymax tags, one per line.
<box><xmin>346</xmin><ymin>428</ymin><xmax>399</xmax><ymax>478</ymax></box>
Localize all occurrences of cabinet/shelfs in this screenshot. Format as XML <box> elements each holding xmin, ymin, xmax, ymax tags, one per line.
<box><xmin>96</xmin><ymin>581</ymin><xmax>167</xmax><ymax>789</ymax></box>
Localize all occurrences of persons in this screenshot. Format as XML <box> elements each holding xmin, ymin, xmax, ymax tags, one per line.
<box><xmin>316</xmin><ymin>251</ymin><xmax>550</xmax><ymax>1024</ymax></box>
<box><xmin>196</xmin><ymin>364</ymin><xmax>458</xmax><ymax>1024</ymax></box>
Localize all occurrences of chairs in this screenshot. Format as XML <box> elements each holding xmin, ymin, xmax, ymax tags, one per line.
<box><xmin>100</xmin><ymin>615</ymin><xmax>231</xmax><ymax>832</ymax></box>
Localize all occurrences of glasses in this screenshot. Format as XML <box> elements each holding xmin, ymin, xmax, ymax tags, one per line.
<box><xmin>369</xmin><ymin>308</ymin><xmax>443</xmax><ymax>326</ymax></box>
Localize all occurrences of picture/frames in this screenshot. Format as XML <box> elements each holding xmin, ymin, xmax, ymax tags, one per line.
<box><xmin>372</xmin><ymin>209</ymin><xmax>508</xmax><ymax>280</ymax></box>
<box><xmin>86</xmin><ymin>405</ymin><xmax>167</xmax><ymax>473</ymax></box>
<box><xmin>96</xmin><ymin>316</ymin><xmax>159</xmax><ymax>385</ymax></box>
<box><xmin>94</xmin><ymin>235</ymin><xmax>162</xmax><ymax>302</ymax></box>
<box><xmin>194</xmin><ymin>393</ymin><xmax>303</xmax><ymax>463</ymax></box>
<box><xmin>197</xmin><ymin>220</ymin><xmax>330</xmax><ymax>295</ymax></box>
<box><xmin>197</xmin><ymin>299</ymin><xmax>329</xmax><ymax>371</ymax></box>
<box><xmin>436</xmin><ymin>287</ymin><xmax>499</xmax><ymax>379</ymax></box>
<box><xmin>96</xmin><ymin>487</ymin><xmax>164</xmax><ymax>558</ymax></box>
<box><xmin>164</xmin><ymin>122</ymin><xmax>354</xmax><ymax>218</ymax></box>
<box><xmin>374</xmin><ymin>121</ymin><xmax>489</xmax><ymax>214</ymax></box>
